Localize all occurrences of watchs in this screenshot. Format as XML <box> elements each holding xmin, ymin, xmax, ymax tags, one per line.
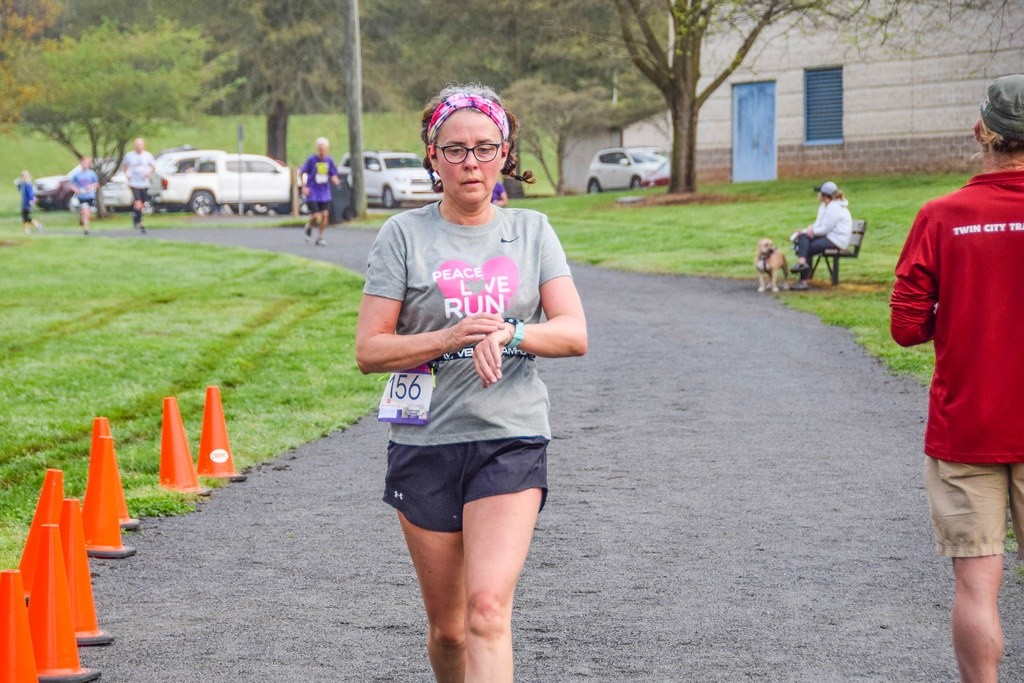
<box><xmin>504</xmin><ymin>318</ymin><xmax>524</xmax><ymax>348</ymax></box>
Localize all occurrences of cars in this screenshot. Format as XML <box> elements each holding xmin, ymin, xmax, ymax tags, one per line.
<box><xmin>586</xmin><ymin>144</ymin><xmax>672</xmax><ymax>194</ymax></box>
<box><xmin>33</xmin><ymin>148</ymin><xmax>227</xmax><ymax>217</ymax></box>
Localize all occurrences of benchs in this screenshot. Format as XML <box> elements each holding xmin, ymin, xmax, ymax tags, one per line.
<box><xmin>805</xmin><ymin>219</ymin><xmax>867</xmax><ymax>287</ymax></box>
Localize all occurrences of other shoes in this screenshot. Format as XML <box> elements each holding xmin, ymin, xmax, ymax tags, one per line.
<box><xmin>791</xmin><ymin>284</ymin><xmax>808</xmax><ymax>290</ymax></box>
<box><xmin>790</xmin><ymin>263</ymin><xmax>810</xmax><ymax>272</ymax></box>
<box><xmin>304</xmin><ymin>223</ymin><xmax>312</xmax><ymax>241</ymax></box>
<box><xmin>317</xmin><ymin>239</ymin><xmax>326</xmax><ymax>245</ymax></box>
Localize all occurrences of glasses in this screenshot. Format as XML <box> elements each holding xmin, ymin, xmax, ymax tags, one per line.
<box><xmin>436</xmin><ymin>141</ymin><xmax>502</xmax><ymax>163</ymax></box>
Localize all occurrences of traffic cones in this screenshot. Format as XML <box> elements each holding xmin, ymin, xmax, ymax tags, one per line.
<box><xmin>196</xmin><ymin>386</ymin><xmax>249</xmax><ymax>482</ymax></box>
<box><xmin>159</xmin><ymin>396</ymin><xmax>214</xmax><ymax>497</ymax></box>
<box><xmin>0</xmin><ymin>416</ymin><xmax>141</xmax><ymax>683</ymax></box>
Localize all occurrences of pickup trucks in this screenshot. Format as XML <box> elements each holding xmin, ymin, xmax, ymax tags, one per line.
<box><xmin>151</xmin><ymin>154</ymin><xmax>314</xmax><ymax>218</ymax></box>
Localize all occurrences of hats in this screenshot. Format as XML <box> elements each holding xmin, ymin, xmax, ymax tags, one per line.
<box><xmin>980</xmin><ymin>73</ymin><xmax>1024</xmax><ymax>137</ymax></box>
<box><xmin>814</xmin><ymin>180</ymin><xmax>837</xmax><ymax>196</ymax></box>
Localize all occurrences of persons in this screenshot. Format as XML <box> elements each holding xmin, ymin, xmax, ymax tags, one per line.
<box><xmin>14</xmin><ymin>171</ymin><xmax>44</xmax><ymax>234</ymax></box>
<box><xmin>299</xmin><ymin>137</ymin><xmax>340</xmax><ymax>247</ymax></box>
<box><xmin>888</xmin><ymin>74</ymin><xmax>1024</xmax><ymax>683</ymax></box>
<box><xmin>120</xmin><ymin>138</ymin><xmax>157</xmax><ymax>231</ymax></box>
<box><xmin>491</xmin><ymin>181</ymin><xmax>508</xmax><ymax>207</ymax></box>
<box><xmin>68</xmin><ymin>156</ymin><xmax>99</xmax><ymax>235</ymax></box>
<box><xmin>355</xmin><ymin>84</ymin><xmax>587</xmax><ymax>683</ymax></box>
<box><xmin>790</xmin><ymin>181</ymin><xmax>852</xmax><ymax>291</ymax></box>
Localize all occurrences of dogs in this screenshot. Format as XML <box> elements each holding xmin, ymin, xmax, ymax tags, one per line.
<box><xmin>754</xmin><ymin>238</ymin><xmax>789</xmax><ymax>293</ymax></box>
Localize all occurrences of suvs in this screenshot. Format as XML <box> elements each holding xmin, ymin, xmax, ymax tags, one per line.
<box><xmin>335</xmin><ymin>148</ymin><xmax>445</xmax><ymax>209</ymax></box>
